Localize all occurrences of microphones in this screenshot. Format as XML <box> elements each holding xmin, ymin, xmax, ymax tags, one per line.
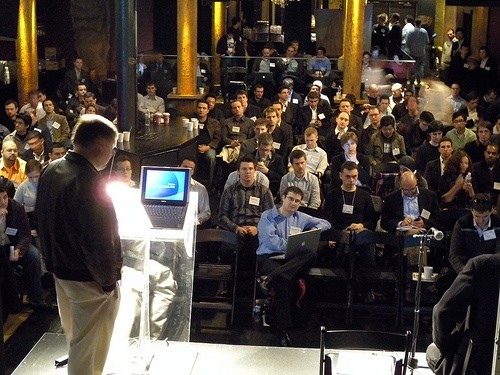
<box><xmin>431</xmin><ymin>227</ymin><xmax>444</xmax><ymax>241</ymax></box>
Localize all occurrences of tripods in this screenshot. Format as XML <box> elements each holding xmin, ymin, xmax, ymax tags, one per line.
<box><xmin>402</xmin><ymin>234</ymin><xmax>434</xmax><ymax>375</ymax></box>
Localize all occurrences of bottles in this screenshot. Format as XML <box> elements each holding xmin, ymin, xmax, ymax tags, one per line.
<box><xmin>145</xmin><ymin>109</ymin><xmax>150</xmax><ymax>127</ymax></box>
<box><xmin>38</xmin><ymin>61</ymin><xmax>46</xmax><ymax>74</ymax></box>
<box><xmin>253</xmin><ymin>299</ymin><xmax>262</xmax><ymax>329</ymax></box>
<box><xmin>464</xmin><ymin>172</ymin><xmax>472</xmax><ymax>184</ymax></box>
<box><xmin>4</xmin><ymin>67</ymin><xmax>10</xmax><ymax>85</ymax></box>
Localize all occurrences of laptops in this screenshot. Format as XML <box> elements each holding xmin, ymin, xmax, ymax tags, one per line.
<box><xmin>269</xmin><ymin>228</ymin><xmax>321</xmax><ymax>260</ymax></box>
<box><xmin>139</xmin><ymin>167</ymin><xmax>192</xmax><ymax>230</ymax></box>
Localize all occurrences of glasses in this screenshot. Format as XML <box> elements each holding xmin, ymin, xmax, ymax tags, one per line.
<box><xmin>14</xmin><ymin>121</ymin><xmax>24</xmax><ymax>125</ymax></box>
<box><xmin>116</xmin><ymin>168</ymin><xmax>132</xmax><ymax>173</ymax></box>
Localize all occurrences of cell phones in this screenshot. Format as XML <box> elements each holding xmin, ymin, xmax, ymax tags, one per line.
<box><xmin>414</xmin><ymin>218</ymin><xmax>421</xmax><ymax>221</ymax></box>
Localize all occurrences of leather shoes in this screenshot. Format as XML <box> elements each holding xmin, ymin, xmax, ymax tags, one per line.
<box><xmin>280</xmin><ymin>329</ymin><xmax>294</xmax><ymax>346</ymax></box>
<box><xmin>257</xmin><ymin>274</ymin><xmax>273</xmax><ymax>295</ymax></box>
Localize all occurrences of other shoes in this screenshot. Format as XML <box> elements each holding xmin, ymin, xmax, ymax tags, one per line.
<box><xmin>403</xmin><ymin>288</ymin><xmax>415</xmax><ymax>306</ymax></box>
<box><xmin>31</xmin><ymin>300</ymin><xmax>49</xmax><ymax>310</ymax></box>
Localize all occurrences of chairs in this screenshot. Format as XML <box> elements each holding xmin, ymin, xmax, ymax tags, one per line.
<box><xmin>192</xmin><ymin>229</ymin><xmax>455</xmax><ymax>331</ymax></box>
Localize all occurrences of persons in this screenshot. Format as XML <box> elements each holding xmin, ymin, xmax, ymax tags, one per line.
<box><xmin>426</xmin><ymin>252</ymin><xmax>500</xmax><ymax>375</ymax></box>
<box><xmin>34</xmin><ymin>113</ymin><xmax>123</xmax><ymax>375</ymax></box>
<box><xmin>0</xmin><ymin>14</ymin><xmax>500</xmax><ymax>346</ymax></box>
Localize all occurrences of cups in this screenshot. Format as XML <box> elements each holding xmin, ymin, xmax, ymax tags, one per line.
<box><xmin>423</xmin><ymin>266</ymin><xmax>433</xmax><ymax>278</ymax></box>
<box><xmin>122</xmin><ymin>132</ymin><xmax>130</xmax><ymax>142</ymax></box>
<box><xmin>173</xmin><ymin>88</ymin><xmax>177</xmax><ymax>94</ymax></box>
<box><xmin>151</xmin><ymin>112</ymin><xmax>170</xmax><ymax>124</ymax></box>
<box><xmin>181</xmin><ymin>118</ymin><xmax>198</xmax><ymax>132</ymax></box>
<box><xmin>336</xmin><ymin>92</ymin><xmax>346</xmax><ymax>99</ymax></box>
<box><xmin>199</xmin><ymin>88</ymin><xmax>204</xmax><ymax>95</ymax></box>
<box><xmin>118</xmin><ymin>133</ymin><xmax>124</xmax><ymax>142</ymax></box>
